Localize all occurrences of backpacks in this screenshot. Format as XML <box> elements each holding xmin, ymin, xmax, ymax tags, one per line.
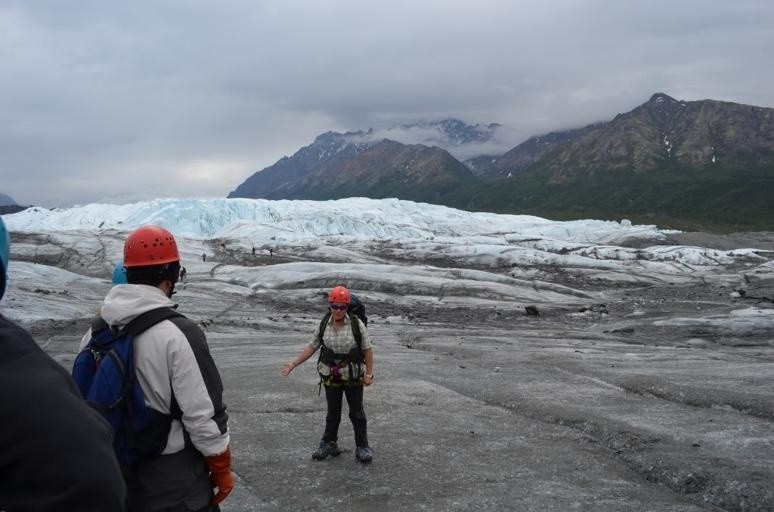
<box><xmin>319</xmin><ymin>294</ymin><xmax>368</xmax><ymax>346</ymax></box>
<box><xmin>71</xmin><ymin>305</ymin><xmax>187</xmax><ymax>466</ymax></box>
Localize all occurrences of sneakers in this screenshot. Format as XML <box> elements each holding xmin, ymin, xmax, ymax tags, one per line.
<box><xmin>312</xmin><ymin>440</ymin><xmax>339</xmax><ymax>460</ymax></box>
<box><xmin>355</xmin><ymin>446</ymin><xmax>372</xmax><ymax>461</ymax></box>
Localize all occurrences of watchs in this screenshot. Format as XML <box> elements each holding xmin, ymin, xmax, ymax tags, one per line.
<box><xmin>365</xmin><ymin>374</ymin><xmax>374</xmax><ymax>379</ymax></box>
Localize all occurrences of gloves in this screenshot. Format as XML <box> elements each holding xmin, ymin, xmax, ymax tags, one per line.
<box><xmin>209</xmin><ymin>446</ymin><xmax>235</xmax><ymax>506</ymax></box>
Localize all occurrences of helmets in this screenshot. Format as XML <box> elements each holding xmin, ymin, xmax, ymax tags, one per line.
<box><xmin>123</xmin><ymin>225</ymin><xmax>180</xmax><ymax>269</ymax></box>
<box><xmin>328</xmin><ymin>286</ymin><xmax>350</xmax><ymax>304</ymax></box>
<box><xmin>0</xmin><ymin>218</ymin><xmax>9</xmax><ymax>300</ymax></box>
<box><xmin>112</xmin><ymin>261</ymin><xmax>127</xmax><ymax>284</ymax></box>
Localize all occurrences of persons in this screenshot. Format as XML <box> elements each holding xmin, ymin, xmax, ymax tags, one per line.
<box><xmin>250</xmin><ymin>245</ymin><xmax>257</xmax><ymax>257</ymax></box>
<box><xmin>279</xmin><ymin>284</ymin><xmax>375</xmax><ymax>463</ymax></box>
<box><xmin>270</xmin><ymin>248</ymin><xmax>275</xmax><ymax>256</ymax></box>
<box><xmin>0</xmin><ymin>213</ymin><xmax>130</xmax><ymax>512</ymax></box>
<box><xmin>112</xmin><ymin>261</ymin><xmax>129</xmax><ymax>287</ymax></box>
<box><xmin>180</xmin><ymin>270</ymin><xmax>184</xmax><ymax>282</ymax></box>
<box><xmin>182</xmin><ymin>266</ymin><xmax>186</xmax><ymax>278</ymax></box>
<box><xmin>76</xmin><ymin>225</ymin><xmax>235</xmax><ymax>511</ymax></box>
<box><xmin>201</xmin><ymin>252</ymin><xmax>206</xmax><ymax>263</ymax></box>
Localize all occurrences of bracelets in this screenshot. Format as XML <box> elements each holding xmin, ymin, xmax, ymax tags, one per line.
<box><xmin>292</xmin><ymin>362</ymin><xmax>297</xmax><ymax>367</ymax></box>
